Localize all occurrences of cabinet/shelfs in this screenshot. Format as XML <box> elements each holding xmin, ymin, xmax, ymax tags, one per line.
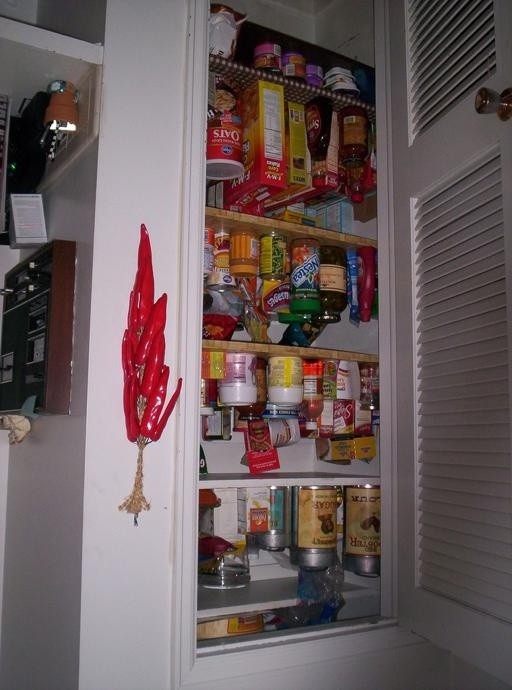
<box><xmin>0</xmin><ymin>238</ymin><xmax>76</xmax><ymax>416</ymax></box>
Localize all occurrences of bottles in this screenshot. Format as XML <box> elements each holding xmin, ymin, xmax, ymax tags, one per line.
<box><xmin>202</xmin><ymin>215</ymin><xmax>379</xmax><ymax>345</ymax></box>
<box><xmin>196</xmin><ymin>589</ymin><xmax>265</xmax><ymax>646</ymax></box>
<box><xmin>206</xmin><ymin>70</ymin><xmax>378</xmax><ymax>225</ymax></box>
<box><xmin>198</xmin><ymin>344</ymin><xmax>379</xmax><ymax>476</ymax></box>
<box><xmin>206</xmin><ymin>4</ymin><xmax>377</xmax><ymax>108</ymax></box>
<box><xmin>199</xmin><ymin>477</ymin><xmax>379</xmax><ymax>589</ymax></box>
